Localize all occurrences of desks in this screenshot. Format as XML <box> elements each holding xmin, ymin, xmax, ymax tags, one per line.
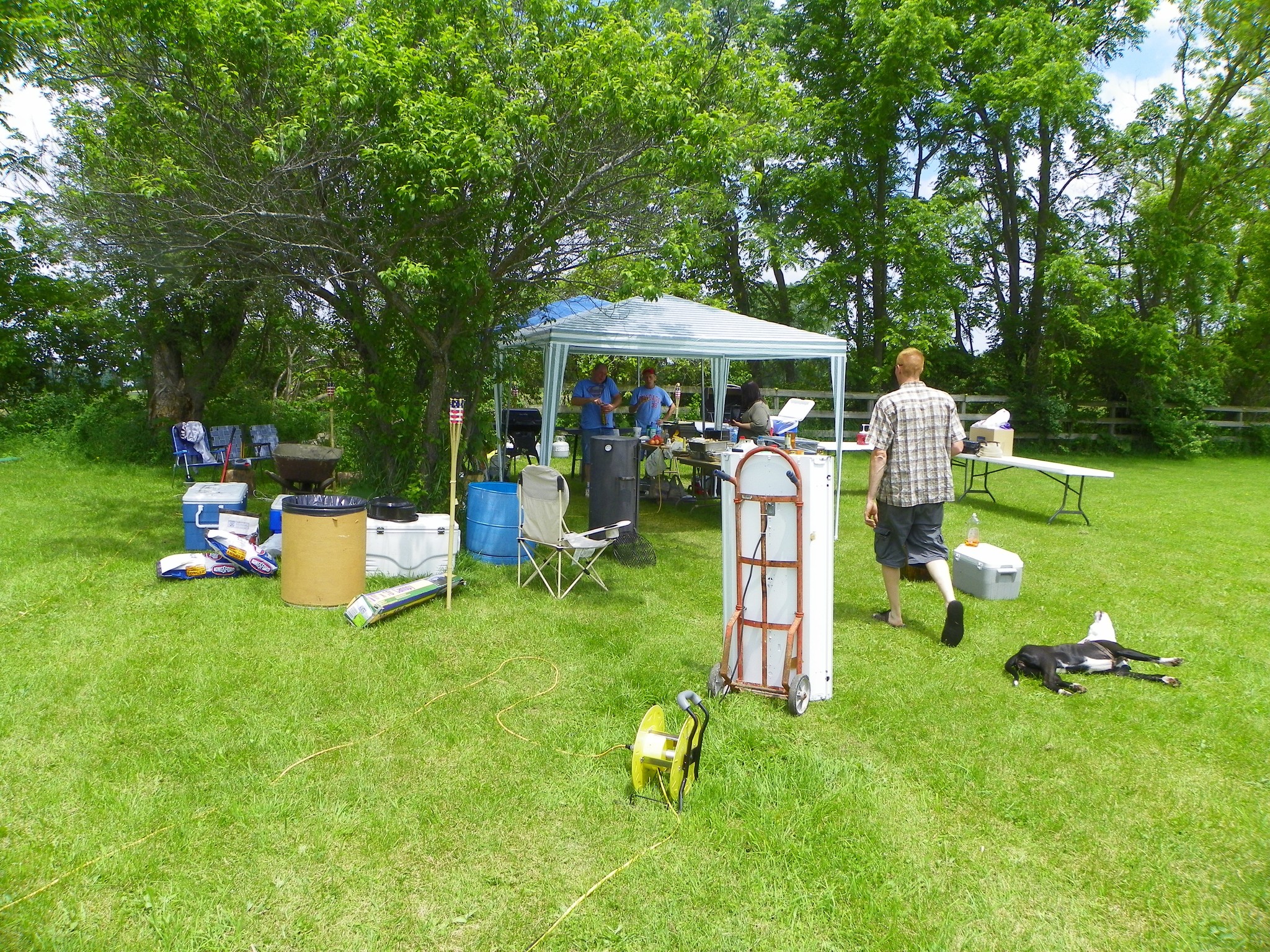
<box><xmin>638</xmin><ymin>441</ymin><xmax>722</xmax><ymax>508</ymax></box>
<box><xmin>554</xmin><ymin>426</ymin><xmax>634</xmax><ymax>484</ymax></box>
<box><xmin>817</xmin><ymin>441</ymin><xmax>875</xmax><ymax>457</ymax></box>
<box><xmin>677</xmin><ymin>455</ymin><xmax>721</xmax><ymax>516</ymax></box>
<box><xmin>950</xmin><ymin>453</ymin><xmax>1114</xmax><ymax>527</ymax></box>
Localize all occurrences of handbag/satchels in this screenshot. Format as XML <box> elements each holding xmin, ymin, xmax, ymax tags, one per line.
<box><xmin>668</xmin><ymin>475</ymin><xmax>685</xmax><ymax>499</ymax></box>
<box><xmin>645</xmin><ymin>447</ymin><xmax>667</xmax><ymax>476</ymax></box>
<box><xmin>649</xmin><ymin>475</ymin><xmax>670</xmax><ymax>500</ymax></box>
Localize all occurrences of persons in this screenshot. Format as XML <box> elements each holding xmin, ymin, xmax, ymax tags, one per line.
<box><xmin>570</xmin><ymin>363</ymin><xmax>622</xmax><ymax>497</ymax></box>
<box><xmin>628</xmin><ymin>367</ymin><xmax>677</xmax><ymax>479</ymax></box>
<box><xmin>863</xmin><ymin>348</ymin><xmax>967</xmax><ymax>648</ymax></box>
<box><xmin>729</xmin><ymin>380</ymin><xmax>771</xmax><ymax>444</ymax></box>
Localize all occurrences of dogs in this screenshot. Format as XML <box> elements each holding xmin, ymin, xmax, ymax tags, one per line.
<box><xmin>1002</xmin><ymin>611</ymin><xmax>1184</xmax><ymax>696</ymax></box>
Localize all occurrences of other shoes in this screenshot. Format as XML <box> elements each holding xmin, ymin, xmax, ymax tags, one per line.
<box><xmin>585</xmin><ymin>488</ymin><xmax>590</xmax><ymax>497</ymax></box>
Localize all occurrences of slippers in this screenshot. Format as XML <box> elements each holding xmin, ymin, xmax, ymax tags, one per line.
<box><xmin>873</xmin><ymin>610</ymin><xmax>905</xmax><ymax>627</ymax></box>
<box><xmin>941</xmin><ymin>601</ymin><xmax>964</xmax><ymax>648</ymax></box>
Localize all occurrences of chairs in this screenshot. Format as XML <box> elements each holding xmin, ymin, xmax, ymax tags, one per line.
<box><xmin>171</xmin><ymin>420</ymin><xmax>227</xmax><ymax>489</ymax></box>
<box><xmin>249</xmin><ymin>424</ymin><xmax>288</xmax><ymax>474</ymax></box>
<box><xmin>515</xmin><ymin>464</ymin><xmax>632</xmax><ymax>601</ymax></box>
<box><xmin>210</xmin><ymin>424</ymin><xmax>265</xmax><ymax>480</ymax></box>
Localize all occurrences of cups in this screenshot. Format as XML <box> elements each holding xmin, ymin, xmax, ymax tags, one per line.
<box><xmin>633</xmin><ymin>427</ymin><xmax>642</xmax><ymax>438</ymax></box>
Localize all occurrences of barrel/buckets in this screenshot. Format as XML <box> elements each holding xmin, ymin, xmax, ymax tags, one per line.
<box><xmin>466</xmin><ymin>481</ymin><xmax>535</xmax><ymax>565</ymax></box>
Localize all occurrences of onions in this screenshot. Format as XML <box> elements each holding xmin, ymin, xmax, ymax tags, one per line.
<box><xmin>650</xmin><ymin>435</ymin><xmax>664</xmax><ymax>445</ymax></box>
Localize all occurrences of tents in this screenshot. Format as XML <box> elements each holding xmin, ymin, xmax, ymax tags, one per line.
<box><xmin>489</xmin><ymin>291</ymin><xmax>846</xmax><ymax>539</ymax></box>
<box><xmin>478</xmin><ymin>296</ymin><xmax>705</xmax><ymax>443</ymax></box>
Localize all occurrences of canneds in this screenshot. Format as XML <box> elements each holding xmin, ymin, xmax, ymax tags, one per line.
<box><xmin>784</xmin><ymin>431</ymin><xmax>796</xmax><ymax>449</ymax></box>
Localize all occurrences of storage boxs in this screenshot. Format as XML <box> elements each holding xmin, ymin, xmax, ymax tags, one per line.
<box><xmin>269</xmin><ymin>494</ymin><xmax>296</xmax><ymax>534</ymax></box>
<box><xmin>365</xmin><ymin>512</ymin><xmax>461</xmax><ymax>579</ymax></box>
<box><xmin>968</xmin><ymin>425</ymin><xmax>1014</xmax><ymax>456</ymax></box>
<box><xmin>951</xmin><ymin>542</ymin><xmax>1025</xmax><ymax>601</ymax></box>
<box><xmin>181</xmin><ymin>481</ymin><xmax>248</xmax><ymax>550</ymax></box>
<box><xmin>769</xmin><ymin>398</ymin><xmax>816</xmax><ymax>437</ymax></box>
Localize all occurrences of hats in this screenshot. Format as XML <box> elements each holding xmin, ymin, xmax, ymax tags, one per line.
<box><xmin>641</xmin><ymin>367</ymin><xmax>655</xmax><ymax>375</ymax></box>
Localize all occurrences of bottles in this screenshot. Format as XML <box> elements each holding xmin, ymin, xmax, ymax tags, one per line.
<box><xmin>757</xmin><ymin>436</ymin><xmax>764</xmax><ymax>447</ymax></box>
<box><xmin>964</xmin><ymin>512</ymin><xmax>981</xmax><ymax>547</ymax></box>
<box><xmin>661</xmin><ymin>428</ymin><xmax>670</xmax><ymax>444</ymax></box>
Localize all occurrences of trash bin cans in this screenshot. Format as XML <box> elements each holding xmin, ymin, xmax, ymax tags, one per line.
<box><xmin>281</xmin><ymin>494</ymin><xmax>369</xmax><ymax>608</ymax></box>
<box><xmin>465</xmin><ymin>481</ymin><xmax>539</xmax><ymax>564</ymax></box>
